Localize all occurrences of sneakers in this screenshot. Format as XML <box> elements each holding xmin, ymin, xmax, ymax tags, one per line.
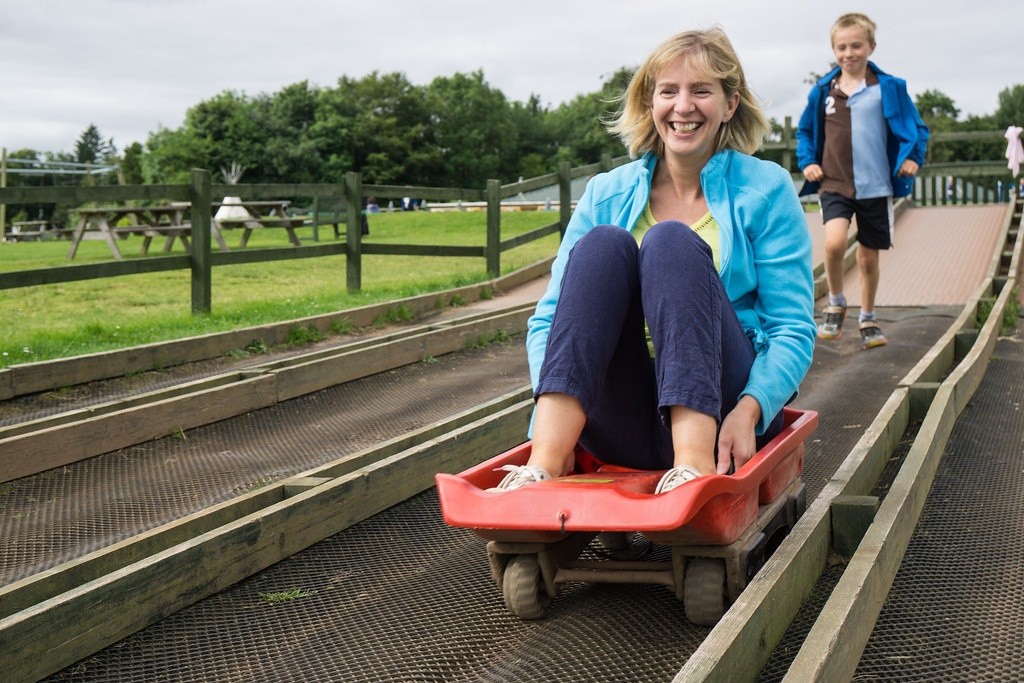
<box><xmin>485</xmin><ymin>463</ymin><xmax>552</xmax><ymax>494</ymax></box>
<box><xmin>818</xmin><ymin>300</ymin><xmax>846</xmax><ymax>338</ymax></box>
<box><xmin>859</xmin><ymin>320</ymin><xmax>888</xmax><ymax>349</ymax></box>
<box><xmin>656</xmin><ymin>465</ymin><xmax>703</xmax><ymax>494</ymax></box>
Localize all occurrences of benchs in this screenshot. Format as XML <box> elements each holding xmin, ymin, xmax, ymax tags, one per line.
<box><xmin>5</xmin><ymin>231</ymin><xmax>43</xmax><ymax>238</ymax></box>
<box><xmin>214</xmin><ymin>216</ymin><xmax>307</xmax><ymax>232</ymax></box>
<box><xmin>105</xmin><ymin>223</ymin><xmax>192</xmax><ymax>241</ymax></box>
<box><xmin>303</xmin><ymin>195</ymin><xmax>349</xmax><ymax>242</ymax></box>
<box><xmin>49</xmin><ymin>225</ymin><xmax>145</xmax><ymax>242</ymax></box>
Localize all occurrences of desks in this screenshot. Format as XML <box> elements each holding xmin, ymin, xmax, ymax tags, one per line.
<box><xmin>163</xmin><ymin>200</ymin><xmax>302</xmax><ymax>252</ymax></box>
<box><xmin>65</xmin><ymin>204</ymin><xmax>190</xmax><ymax>260</ymax></box>
<box><xmin>10</xmin><ymin>220</ymin><xmax>59</xmax><ymax>243</ymax></box>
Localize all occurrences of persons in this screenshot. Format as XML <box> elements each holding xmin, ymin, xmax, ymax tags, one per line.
<box><xmin>366</xmin><ymin>196</ymin><xmax>380</xmax><ymax>214</ymax></box>
<box><xmin>794</xmin><ymin>14</ymin><xmax>930</xmax><ymax>350</ymax></box>
<box><xmin>403</xmin><ymin>198</ymin><xmax>418</xmax><ymax>210</ymax></box>
<box><xmin>485</xmin><ymin>25</ymin><xmax>818</xmax><ymax>494</ymax></box>
